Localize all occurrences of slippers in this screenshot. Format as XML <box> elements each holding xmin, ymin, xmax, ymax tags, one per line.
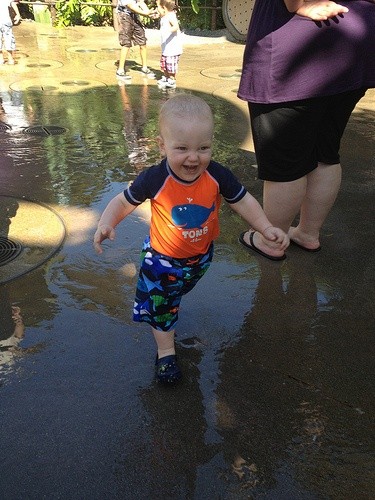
<box><xmin>289</xmin><ymin>236</ymin><xmax>320</xmax><ymax>253</ymax></box>
<box><xmin>238</xmin><ymin>229</ymin><xmax>286</xmax><ymax>261</ymax></box>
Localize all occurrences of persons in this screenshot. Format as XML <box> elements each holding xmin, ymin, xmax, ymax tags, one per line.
<box><xmin>94</xmin><ymin>95</ymin><xmax>290</xmax><ymax>384</ymax></box>
<box><xmin>155</xmin><ymin>0</ymin><xmax>182</xmax><ymax>88</ymax></box>
<box><xmin>237</xmin><ymin>0</ymin><xmax>375</xmax><ymax>260</ymax></box>
<box><xmin>116</xmin><ymin>0</ymin><xmax>160</xmax><ymax>76</ymax></box>
<box><xmin>0</xmin><ymin>0</ymin><xmax>21</xmax><ymax>65</ymax></box>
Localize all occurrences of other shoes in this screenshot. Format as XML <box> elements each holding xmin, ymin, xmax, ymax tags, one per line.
<box><xmin>154</xmin><ymin>344</ymin><xmax>181</xmax><ymax>384</ymax></box>
<box><xmin>164</xmin><ymin>78</ymin><xmax>175</xmax><ymax>88</ymax></box>
<box><xmin>156</xmin><ymin>76</ymin><xmax>168</xmax><ymax>85</ymax></box>
<box><xmin>139</xmin><ymin>68</ymin><xmax>156</xmax><ymax>75</ymax></box>
<box><xmin>115</xmin><ymin>71</ymin><xmax>131</xmax><ymax>80</ymax></box>
